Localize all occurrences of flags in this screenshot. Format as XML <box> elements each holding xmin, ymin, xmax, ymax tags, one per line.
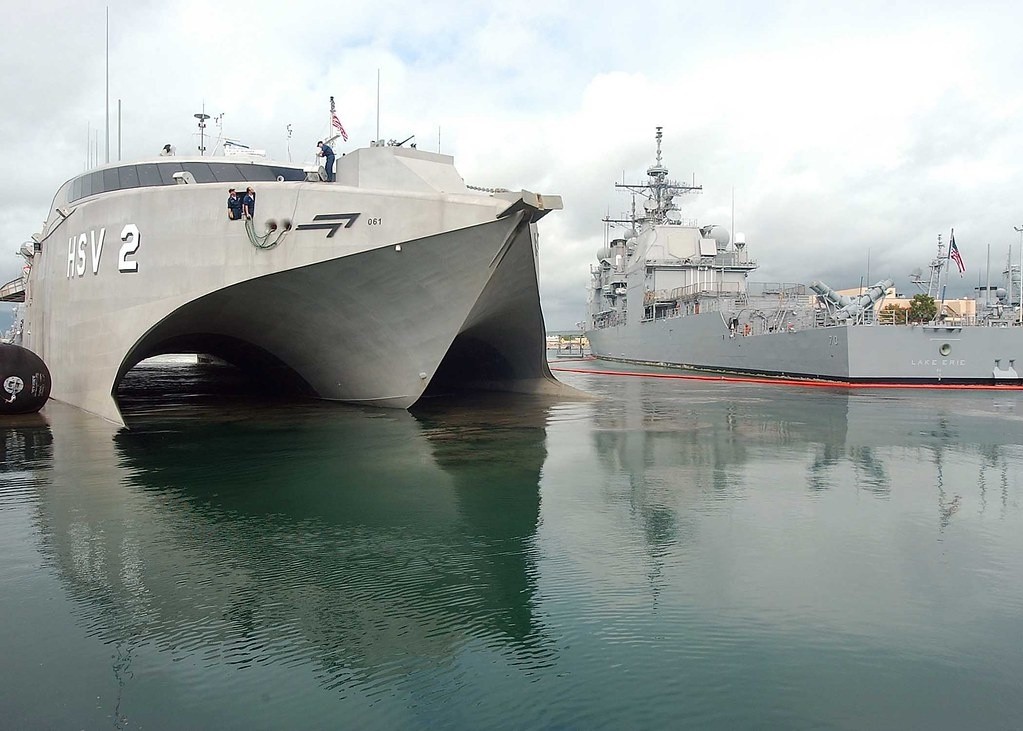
<box><xmin>948</xmin><ymin>228</ymin><xmax>965</xmax><ymax>278</ymax></box>
<box><xmin>331</xmin><ymin>113</ymin><xmax>349</xmax><ymax>142</ymax></box>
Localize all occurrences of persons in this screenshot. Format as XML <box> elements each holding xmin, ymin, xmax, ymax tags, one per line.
<box><xmin>317</xmin><ymin>141</ymin><xmax>335</xmax><ymax>182</ymax></box>
<box><xmin>228</xmin><ymin>189</ymin><xmax>242</xmax><ymax>220</ymax></box>
<box><xmin>243</xmin><ymin>187</ymin><xmax>255</xmax><ymax>219</ymax></box>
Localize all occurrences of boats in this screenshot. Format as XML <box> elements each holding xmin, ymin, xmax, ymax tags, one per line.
<box><xmin>578</xmin><ymin>124</ymin><xmax>1023</xmax><ymax>385</ymax></box>
<box><xmin>0</xmin><ymin>4</ymin><xmax>604</xmax><ymax>433</ymax></box>
